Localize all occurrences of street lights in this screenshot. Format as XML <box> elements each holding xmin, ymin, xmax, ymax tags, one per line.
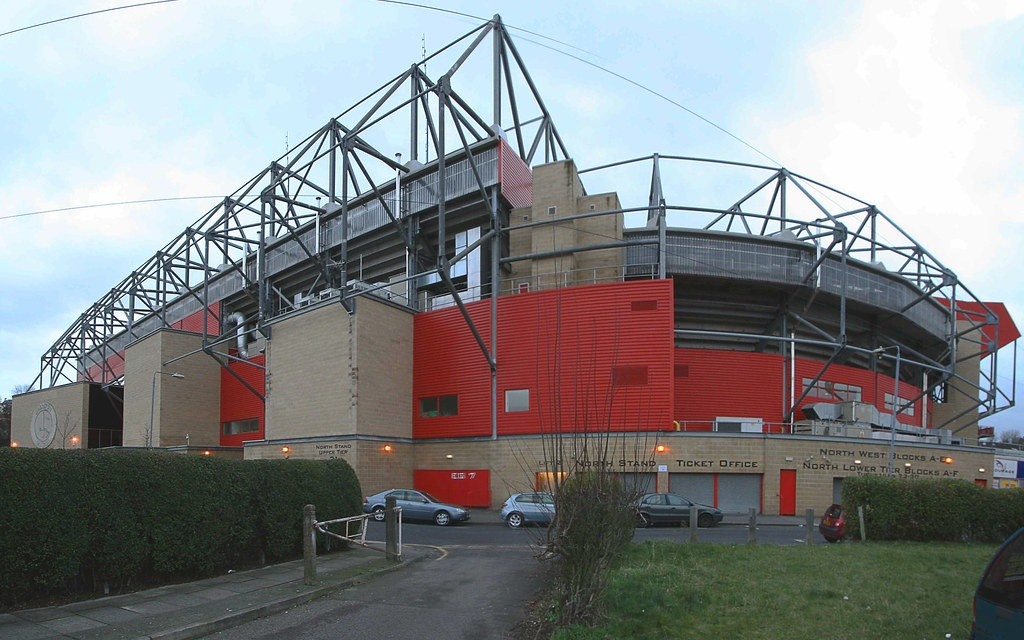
<box><xmin>149</xmin><ymin>371</ymin><xmax>187</xmax><ymax>448</ymax></box>
<box><xmin>875</xmin><ymin>346</ymin><xmax>902</xmax><ymax>479</ymax></box>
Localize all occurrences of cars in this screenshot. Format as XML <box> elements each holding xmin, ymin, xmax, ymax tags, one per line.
<box><xmin>499</xmin><ymin>491</ymin><xmax>555</xmax><ymax>528</ymax></box>
<box><xmin>362</xmin><ymin>488</ymin><xmax>472</xmax><ymax>527</ymax></box>
<box><xmin>820</xmin><ymin>503</ymin><xmax>850</xmax><ymax>544</ymax></box>
<box><xmin>627</xmin><ymin>492</ymin><xmax>723</xmax><ymax>528</ymax></box>
<box><xmin>969</xmin><ymin>525</ymin><xmax>1024</xmax><ymax>640</ymax></box>
